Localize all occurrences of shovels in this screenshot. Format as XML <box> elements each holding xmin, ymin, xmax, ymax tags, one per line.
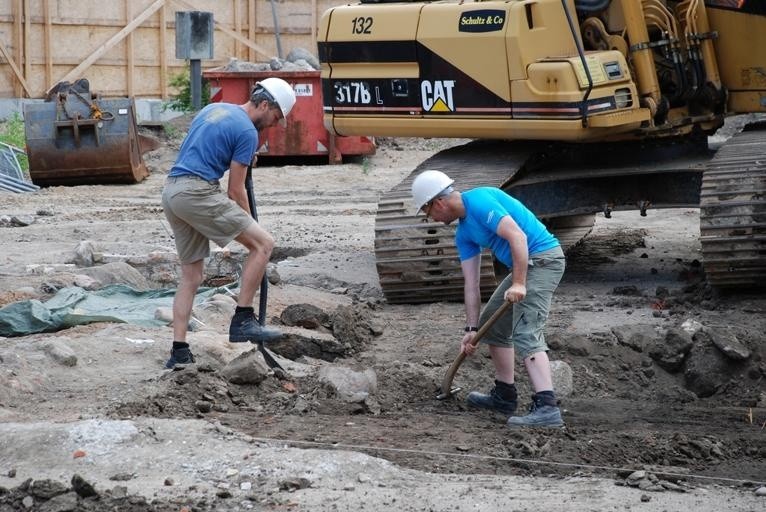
<box><xmin>246</xmin><ymin>161</ymin><xmax>290</xmax><ymax>378</ymax></box>
<box><xmin>436</xmin><ymin>301</ymin><xmax>511</xmax><ymax>400</ymax></box>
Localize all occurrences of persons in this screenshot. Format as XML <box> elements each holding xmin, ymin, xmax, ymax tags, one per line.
<box><xmin>410</xmin><ymin>169</ymin><xmax>566</xmax><ymax>431</ymax></box>
<box><xmin>160</xmin><ymin>78</ymin><xmax>297</xmax><ymax>370</ymax></box>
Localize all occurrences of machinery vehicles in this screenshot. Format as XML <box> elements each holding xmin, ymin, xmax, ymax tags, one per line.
<box><xmin>316</xmin><ymin>2</ymin><xmax>765</xmax><ymax>307</ymax></box>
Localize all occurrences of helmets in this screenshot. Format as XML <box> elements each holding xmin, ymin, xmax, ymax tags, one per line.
<box><xmin>252</xmin><ymin>76</ymin><xmax>300</xmax><ymax>130</ymax></box>
<box><xmin>411</xmin><ymin>169</ymin><xmax>456</xmax><ymax>218</ymax></box>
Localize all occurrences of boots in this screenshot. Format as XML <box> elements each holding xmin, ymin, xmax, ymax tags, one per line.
<box><xmin>227</xmin><ymin>304</ymin><xmax>283</xmax><ymax>344</ymax></box>
<box><xmin>166</xmin><ymin>345</ymin><xmax>195</xmax><ymax>369</ymax></box>
<box><xmin>508</xmin><ymin>392</ymin><xmax>566</xmax><ymax>428</ymax></box>
<box><xmin>467</xmin><ymin>380</ymin><xmax>519</xmax><ymax>413</ymax></box>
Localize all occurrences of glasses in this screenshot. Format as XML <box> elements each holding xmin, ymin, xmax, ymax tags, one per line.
<box><xmin>424</xmin><ymin>201</ymin><xmax>436</xmax><ymax>220</ymax></box>
